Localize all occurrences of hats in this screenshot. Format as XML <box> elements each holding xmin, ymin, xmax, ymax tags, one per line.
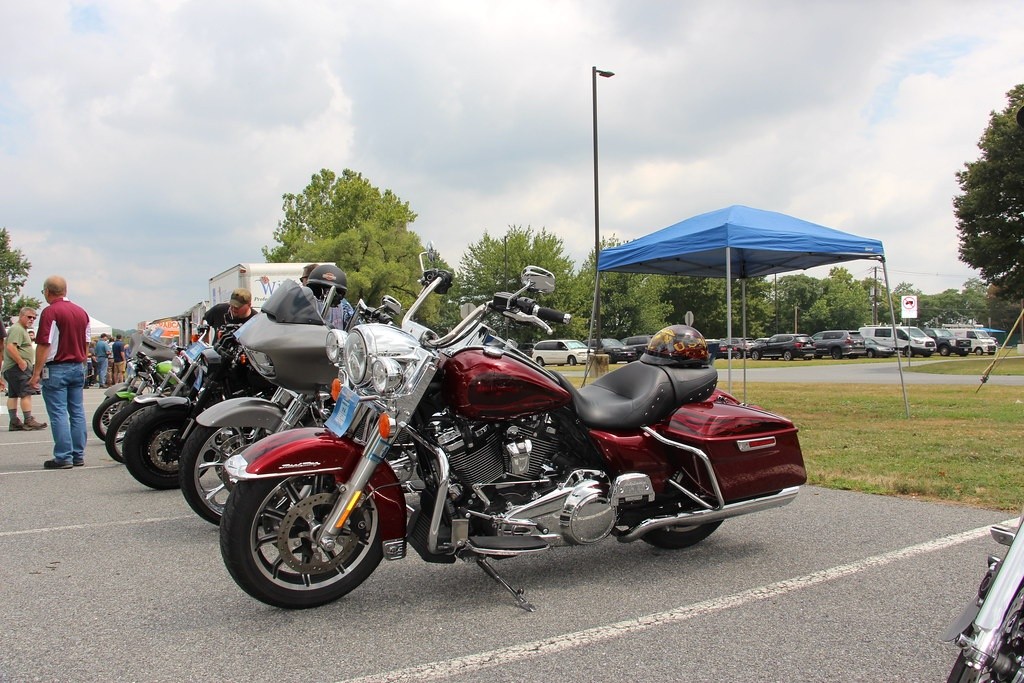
<box><xmin>230</xmin><ymin>288</ymin><xmax>251</xmax><ymax>308</ymax></box>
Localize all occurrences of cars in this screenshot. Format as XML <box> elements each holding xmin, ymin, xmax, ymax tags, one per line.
<box><xmin>582</xmin><ymin>339</ymin><xmax>637</xmax><ymax>364</ymax></box>
<box><xmin>864</xmin><ymin>338</ymin><xmax>895</xmax><ymax>358</ymax></box>
<box><xmin>705</xmin><ymin>341</ymin><xmax>738</xmax><ymax>359</ymax></box>
<box><xmin>517</xmin><ymin>343</ymin><xmax>536</xmax><ymax>357</ymax></box>
<box><xmin>531</xmin><ymin>340</ymin><xmax>595</xmax><ymax>367</ymax></box>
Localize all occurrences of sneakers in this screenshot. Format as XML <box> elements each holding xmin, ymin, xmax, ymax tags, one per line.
<box><xmin>9</xmin><ymin>417</ymin><xmax>23</xmax><ymax>430</ymax></box>
<box><xmin>73</xmin><ymin>460</ymin><xmax>84</xmax><ymax>466</ymax></box>
<box><xmin>100</xmin><ymin>384</ymin><xmax>108</xmax><ymax>389</ymax></box>
<box><xmin>23</xmin><ymin>416</ymin><xmax>47</xmax><ymax>431</ymax></box>
<box><xmin>44</xmin><ymin>459</ymin><xmax>73</xmax><ymax>469</ymax></box>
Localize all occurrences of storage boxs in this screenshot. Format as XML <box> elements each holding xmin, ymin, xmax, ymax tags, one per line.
<box><xmin>667</xmin><ymin>401</ymin><xmax>807</xmax><ymax>500</ymax></box>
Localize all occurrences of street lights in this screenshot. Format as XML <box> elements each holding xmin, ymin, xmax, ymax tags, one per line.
<box><xmin>591</xmin><ymin>66</ymin><xmax>614</xmax><ymax>350</ymax></box>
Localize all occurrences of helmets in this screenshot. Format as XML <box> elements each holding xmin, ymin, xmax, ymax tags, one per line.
<box><xmin>640</xmin><ymin>325</ymin><xmax>711</xmax><ymax>364</ymax></box>
<box><xmin>307</xmin><ymin>265</ymin><xmax>348</xmax><ymax>291</ymax></box>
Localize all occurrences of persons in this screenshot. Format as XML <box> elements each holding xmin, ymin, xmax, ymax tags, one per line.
<box><xmin>196</xmin><ymin>287</ymin><xmax>259</xmax><ymax>363</ymax></box>
<box><xmin>299</xmin><ymin>264</ymin><xmax>362</xmax><ymax>330</ymax></box>
<box><xmin>2</xmin><ymin>308</ymin><xmax>47</xmax><ymax>432</ymax></box>
<box><xmin>28</xmin><ymin>274</ymin><xmax>90</xmax><ymax>469</ymax></box>
<box><xmin>0</xmin><ymin>312</ymin><xmax>134</xmax><ymax>396</ymax></box>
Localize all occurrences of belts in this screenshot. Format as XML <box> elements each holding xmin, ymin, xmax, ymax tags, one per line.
<box><xmin>46</xmin><ymin>361</ymin><xmax>81</xmax><ymax>365</ymax></box>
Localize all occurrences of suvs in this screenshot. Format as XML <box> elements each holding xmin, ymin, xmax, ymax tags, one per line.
<box><xmin>923</xmin><ymin>327</ymin><xmax>972</xmax><ymax>356</ymax></box>
<box><xmin>809</xmin><ymin>330</ymin><xmax>867</xmax><ymax>360</ymax></box>
<box><xmin>749</xmin><ymin>334</ymin><xmax>817</xmax><ymax>361</ymax></box>
<box><xmin>720</xmin><ymin>338</ymin><xmax>771</xmax><ymax>359</ymax></box>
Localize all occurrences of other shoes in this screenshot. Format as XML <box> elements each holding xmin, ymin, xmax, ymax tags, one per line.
<box><xmin>83</xmin><ymin>385</ymin><xmax>89</xmax><ymax>389</ymax></box>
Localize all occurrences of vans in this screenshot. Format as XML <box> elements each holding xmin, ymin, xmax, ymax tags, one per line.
<box><xmin>619</xmin><ymin>335</ymin><xmax>655</xmax><ymax>359</ymax></box>
<box><xmin>858</xmin><ymin>322</ymin><xmax>936</xmax><ymax>357</ymax></box>
<box><xmin>941</xmin><ymin>324</ymin><xmax>997</xmax><ymax>356</ymax></box>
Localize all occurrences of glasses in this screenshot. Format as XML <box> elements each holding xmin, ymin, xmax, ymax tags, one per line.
<box><xmin>300</xmin><ymin>276</ymin><xmax>308</xmax><ymax>282</ymax></box>
<box><xmin>27</xmin><ymin>316</ymin><xmax>36</xmax><ymax>319</ymax></box>
<box><xmin>41</xmin><ymin>288</ymin><xmax>46</xmax><ymax>294</ymax></box>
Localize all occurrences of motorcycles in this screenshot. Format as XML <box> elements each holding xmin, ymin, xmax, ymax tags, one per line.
<box><xmin>92</xmin><ymin>359</ymin><xmax>154</xmax><ymax>443</ymax></box>
<box><xmin>123</xmin><ymin>320</ymin><xmax>270</xmax><ymax>490</ymax></box>
<box><xmin>105</xmin><ymin>333</ymin><xmax>181</xmax><ymax>463</ymax></box>
<box><xmin>941</xmin><ymin>515</ymin><xmax>1024</xmax><ymax>683</ymax></box>
<box><xmin>217</xmin><ymin>264</ymin><xmax>807</xmax><ymax>609</ymax></box>
<box><xmin>101</xmin><ymin>357</ymin><xmax>137</xmax><ymax>432</ymax></box>
<box><xmin>177</xmin><ymin>264</ymin><xmax>352</xmax><ymax>527</ymax></box>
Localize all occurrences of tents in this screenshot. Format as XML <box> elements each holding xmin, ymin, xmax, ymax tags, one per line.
<box><xmin>11</xmin><ymin>314</ymin><xmax>113</xmax><ymax>342</ymax></box>
<box><xmin>582</xmin><ymin>206</ymin><xmax>911</xmax><ymax>417</ymax></box>
